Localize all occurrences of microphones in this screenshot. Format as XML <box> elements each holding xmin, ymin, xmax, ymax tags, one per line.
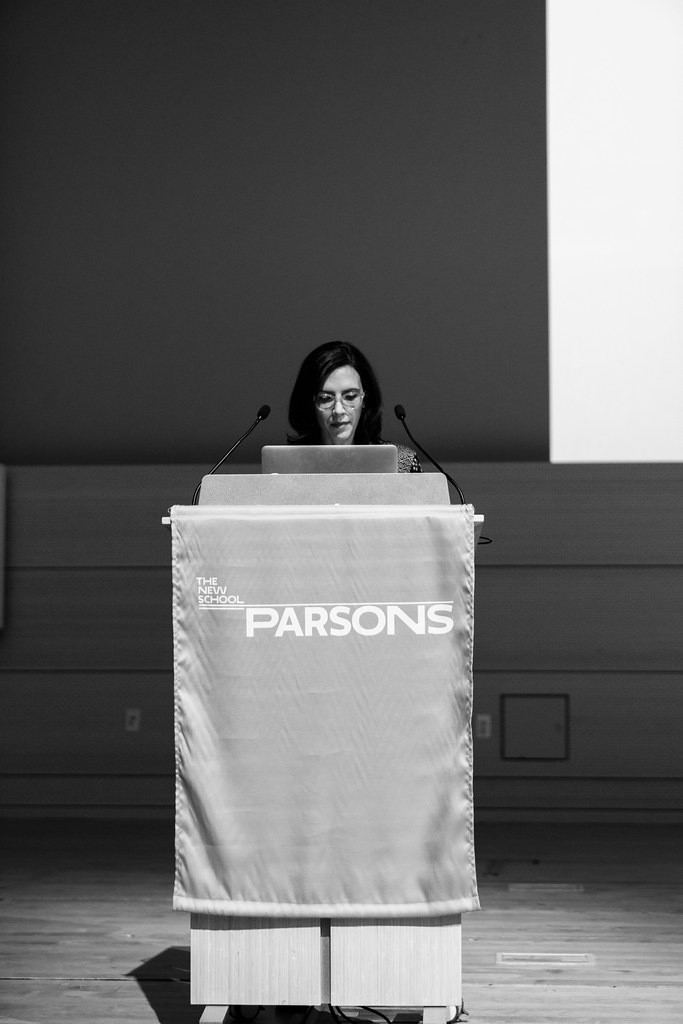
<box><xmin>393</xmin><ymin>404</ymin><xmax>467</xmax><ymax>505</ymax></box>
<box><xmin>191</xmin><ymin>404</ymin><xmax>272</xmax><ymax>505</ymax></box>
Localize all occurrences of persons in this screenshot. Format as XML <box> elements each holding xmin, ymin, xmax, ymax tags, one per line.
<box><xmin>285</xmin><ymin>339</ymin><xmax>423</xmax><ymax>473</ymax></box>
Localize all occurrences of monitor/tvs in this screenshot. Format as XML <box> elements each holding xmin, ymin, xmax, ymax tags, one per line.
<box><xmin>261</xmin><ymin>445</ymin><xmax>399</xmax><ymax>473</ymax></box>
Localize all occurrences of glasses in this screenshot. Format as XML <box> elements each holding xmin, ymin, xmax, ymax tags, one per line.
<box><xmin>314</xmin><ymin>391</ymin><xmax>365</xmax><ymax>412</ymax></box>
